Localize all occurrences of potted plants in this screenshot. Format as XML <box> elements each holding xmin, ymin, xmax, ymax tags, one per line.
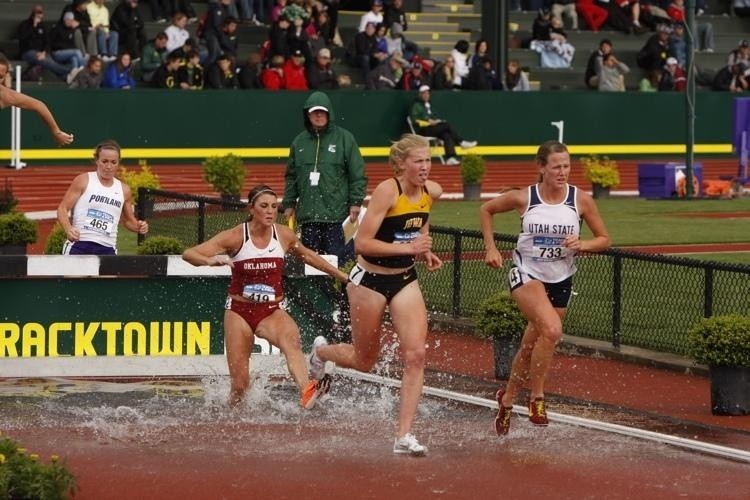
<box><xmin>202</xmin><ymin>152</ymin><xmax>246</xmax><ymax>212</ymax></box>
<box><xmin>474</xmin><ymin>287</ymin><xmax>527</xmax><ymax>377</ymax></box>
<box><xmin>0</xmin><ymin>208</ymin><xmax>38</xmax><ymax>254</ymax></box>
<box><xmin>683</xmin><ymin>311</ymin><xmax>749</xmax><ymax>415</ymax></box>
<box><xmin>581</xmin><ymin>154</ymin><xmax>620</xmax><ymax>200</ymax></box>
<box><xmin>459</xmin><ymin>153</ymin><xmax>485</xmax><ymax>199</ymax></box>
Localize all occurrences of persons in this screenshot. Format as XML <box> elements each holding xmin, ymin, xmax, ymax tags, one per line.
<box><xmin>2</xmin><ymin>1</ymin><xmax>531</xmax><ymax>97</ymax></box>
<box><xmin>1</xmin><ymin>82</ymin><xmax>73</xmax><ymax>147</ymax></box>
<box><xmin>56</xmin><ymin>140</ymin><xmax>148</xmax><ymax>255</ymax></box>
<box><xmin>478</xmin><ymin>141</ymin><xmax>611</xmax><ymax>435</ymax></box>
<box><xmin>280</xmin><ymin>92</ymin><xmax>371</xmax><ymax>308</ymax></box>
<box><xmin>713</xmin><ymin>40</ymin><xmax>750</xmax><ymax>94</ymax></box>
<box><xmin>409</xmin><ymin>85</ymin><xmax>477</xmax><ymax>165</ymax></box>
<box><xmin>182</xmin><ymin>185</ymin><xmax>349</xmax><ymax>410</ymax></box>
<box><xmin>529</xmin><ymin>1</ymin><xmax>714</xmax><ymax>92</ymax></box>
<box><xmin>306</xmin><ymin>134</ymin><xmax>443</xmax><ymax>457</ymax></box>
<box><xmin>730</xmin><ymin>1</ymin><xmax>750</xmax><ymax>18</ymax></box>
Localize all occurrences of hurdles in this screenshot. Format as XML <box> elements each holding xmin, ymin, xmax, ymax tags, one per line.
<box><xmin>0</xmin><ymin>255</ymin><xmax>341</xmax><ymax>379</ymax></box>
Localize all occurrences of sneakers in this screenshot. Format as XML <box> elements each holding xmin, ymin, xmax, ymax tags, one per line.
<box><xmin>393</xmin><ymin>433</ymin><xmax>428</xmax><ymax>454</ymax></box>
<box><xmin>447</xmin><ymin>157</ymin><xmax>461</xmax><ymax>166</ymax></box>
<box><xmin>307</xmin><ymin>335</ymin><xmax>328</xmax><ymax>380</ymax></box>
<box><xmin>527</xmin><ymin>396</ymin><xmax>549</xmax><ymax>426</ymax></box>
<box><xmin>494</xmin><ymin>390</ymin><xmax>513</xmax><ymax>436</ymax></box>
<box><xmin>301</xmin><ymin>373</ymin><xmax>331</xmax><ymax>410</ymax></box>
<box><xmin>461</xmin><ymin>140</ymin><xmax>478</xmax><ymax>150</ymax></box>
